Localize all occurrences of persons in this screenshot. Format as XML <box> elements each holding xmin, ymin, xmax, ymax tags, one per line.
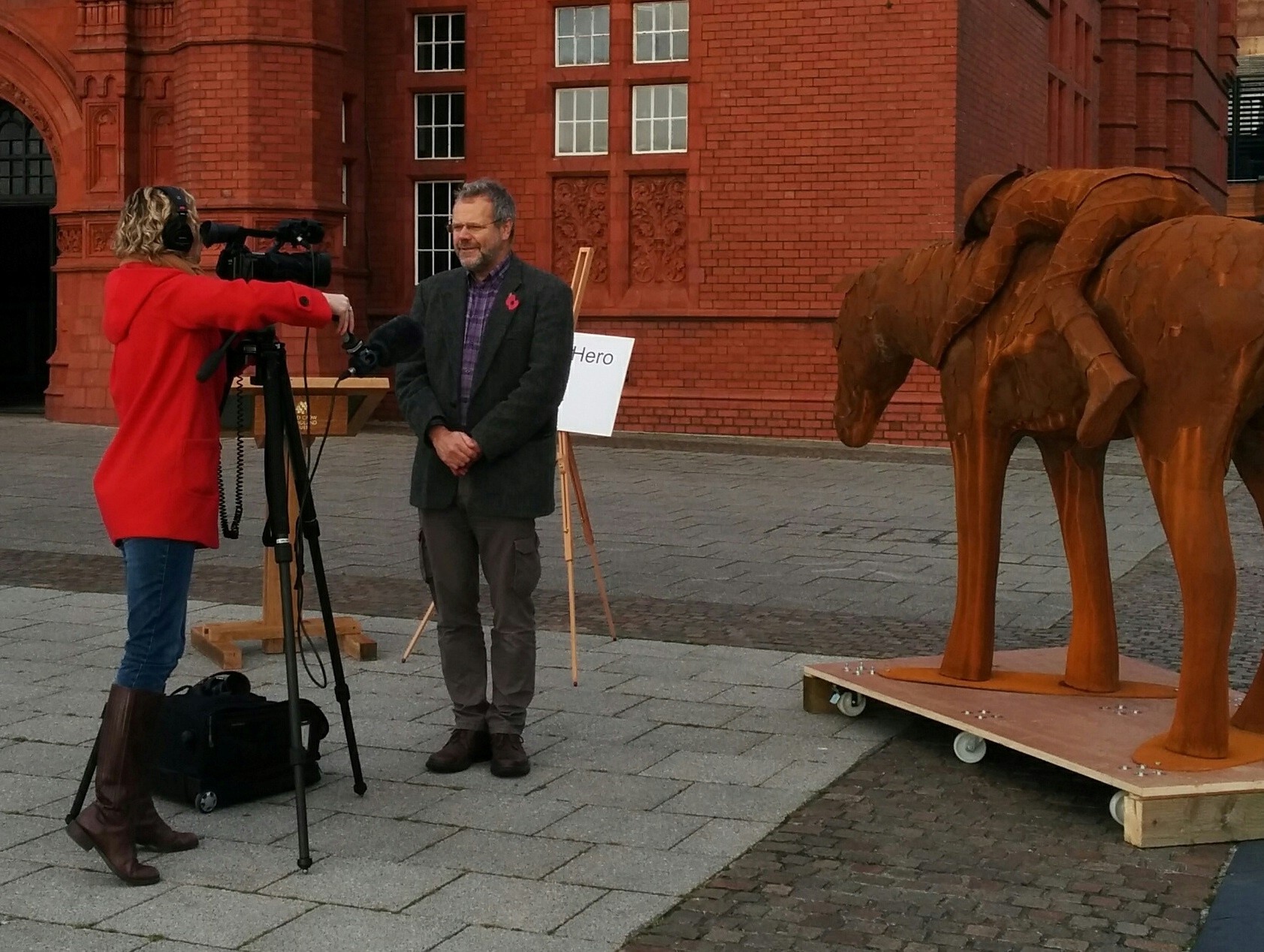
<box><xmin>928</xmin><ymin>168</ymin><xmax>1220</xmax><ymax>448</ymax></box>
<box><xmin>65</xmin><ymin>185</ymin><xmax>355</xmax><ymax>884</ymax></box>
<box><xmin>394</xmin><ymin>181</ymin><xmax>574</xmax><ymax>778</ymax></box>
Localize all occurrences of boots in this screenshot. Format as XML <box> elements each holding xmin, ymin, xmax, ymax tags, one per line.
<box><xmin>130</xmin><ymin>795</ymin><xmax>199</xmax><ymax>852</ymax></box>
<box><xmin>64</xmin><ymin>684</ymin><xmax>160</xmax><ymax>886</ymax></box>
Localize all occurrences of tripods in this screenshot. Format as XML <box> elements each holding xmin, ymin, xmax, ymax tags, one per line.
<box><xmin>66</xmin><ymin>325</ymin><xmax>366</xmax><ymax>873</ymax></box>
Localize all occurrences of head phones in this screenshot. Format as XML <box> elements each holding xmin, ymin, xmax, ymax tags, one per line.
<box><xmin>154</xmin><ymin>186</ymin><xmax>193</xmax><ymax>253</ymax></box>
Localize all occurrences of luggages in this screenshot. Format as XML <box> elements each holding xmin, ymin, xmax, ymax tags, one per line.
<box><xmin>145</xmin><ymin>670</ymin><xmax>330</xmax><ymax>814</ymax></box>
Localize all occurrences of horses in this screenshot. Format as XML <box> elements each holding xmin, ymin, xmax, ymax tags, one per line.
<box><xmin>829</xmin><ymin>217</ymin><xmax>1264</xmax><ymax>757</ymax></box>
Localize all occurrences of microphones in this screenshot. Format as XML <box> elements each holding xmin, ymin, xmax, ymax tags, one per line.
<box><xmin>339</xmin><ymin>314</ymin><xmax>424</xmax><ymax>381</ymax></box>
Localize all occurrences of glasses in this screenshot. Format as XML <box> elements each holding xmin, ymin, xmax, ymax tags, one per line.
<box><xmin>446</xmin><ymin>215</ymin><xmax>513</xmax><ymax>233</ymax></box>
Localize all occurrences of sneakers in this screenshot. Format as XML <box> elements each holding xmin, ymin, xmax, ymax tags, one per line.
<box><xmin>426</xmin><ymin>729</ymin><xmax>490</xmax><ymax>774</ymax></box>
<box><xmin>489</xmin><ymin>733</ymin><xmax>531</xmax><ymax>779</ymax></box>
